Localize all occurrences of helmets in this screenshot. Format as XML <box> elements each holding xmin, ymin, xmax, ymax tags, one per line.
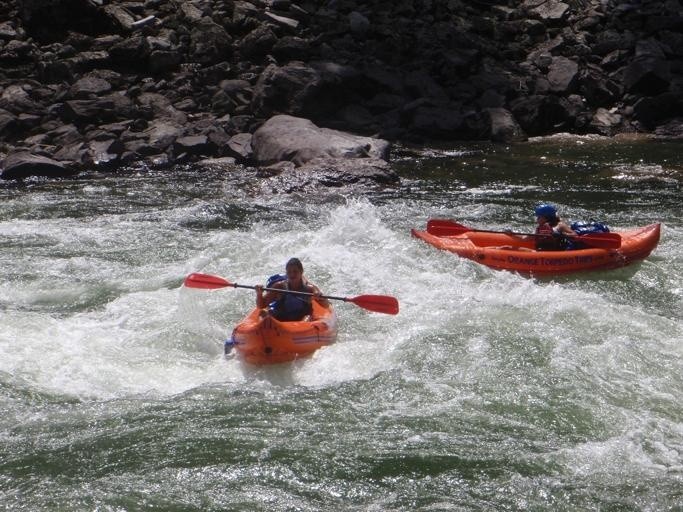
<box><xmin>533</xmin><ymin>204</ymin><xmax>557</xmax><ymax>218</ymax></box>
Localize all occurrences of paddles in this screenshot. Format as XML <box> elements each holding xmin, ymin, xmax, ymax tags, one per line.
<box><xmin>427</xmin><ymin>221</ymin><xmax>622</xmax><ymax>247</ymax></box>
<box><xmin>183</xmin><ymin>274</ymin><xmax>400</xmax><ymax>314</ymax></box>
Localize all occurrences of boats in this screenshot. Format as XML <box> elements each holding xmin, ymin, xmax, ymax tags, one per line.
<box><xmin>410</xmin><ymin>216</ymin><xmax>663</xmax><ymax>276</ymax></box>
<box><xmin>230</xmin><ymin>276</ymin><xmax>340</xmax><ymax>364</ymax></box>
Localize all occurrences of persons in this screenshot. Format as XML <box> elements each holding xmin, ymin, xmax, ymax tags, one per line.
<box><xmin>498</xmin><ymin>204</ymin><xmax>578</xmax><ymax>252</ymax></box>
<box><xmin>255</xmin><ymin>258</ymin><xmax>329</xmax><ymax>322</ymax></box>
